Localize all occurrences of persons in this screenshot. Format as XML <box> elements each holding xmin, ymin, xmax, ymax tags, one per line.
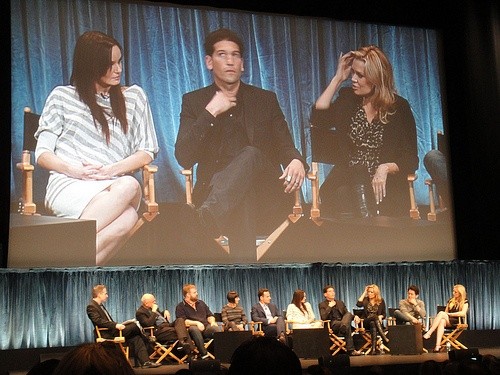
<box><xmin>221</xmin><ymin>290</ymin><xmax>248</xmax><ymax>334</ymax></box>
<box><xmin>175</xmin><ymin>284</ymin><xmax>222</xmax><ymax>362</ymax></box>
<box><xmin>0</xmin><ymin>344</ymin><xmax>500</xmax><ymax>375</ymax></box>
<box><xmin>251</xmin><ymin>287</ymin><xmax>288</xmax><ymax>345</ymax></box>
<box><xmin>304</xmin><ymin>44</ymin><xmax>420</xmax><ymax>224</ymax></box>
<box><xmin>285</xmin><ymin>288</ymin><xmax>325</xmax><ymax>331</ymax></box>
<box><xmin>32</xmin><ymin>30</ymin><xmax>157</xmax><ymax>266</ymax></box>
<box><xmin>422</xmin><ymin>284</ymin><xmax>468</xmax><ymax>353</ymax></box>
<box><xmin>173</xmin><ymin>27</ymin><xmax>309</xmax><ymax>265</ymax></box>
<box><xmin>135</xmin><ymin>294</ymin><xmax>200</xmax><ymax>363</ymax></box>
<box><xmin>393</xmin><ymin>285</ymin><xmax>426</xmax><ymax>327</ymax></box>
<box><xmin>356</xmin><ymin>284</ymin><xmax>389</xmax><ymax>356</ymax></box>
<box><xmin>85</xmin><ymin>285</ymin><xmax>163</xmax><ymax>369</ymax></box>
<box><xmin>318</xmin><ymin>286</ymin><xmax>362</xmax><ymax>356</ymax></box>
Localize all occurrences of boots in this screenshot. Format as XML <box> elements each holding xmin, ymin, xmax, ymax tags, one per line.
<box><xmin>377</xmin><ymin>322</ymin><xmax>389</xmax><ymax>343</ymax></box>
<box><xmin>371</xmin><ymin>328</ymin><xmax>377</xmax><ymax>354</ymax></box>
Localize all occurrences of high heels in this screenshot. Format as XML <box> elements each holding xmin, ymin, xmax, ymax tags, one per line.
<box><xmin>433</xmin><ymin>346</ymin><xmax>442</xmax><ymax>352</ymax></box>
<box><xmin>422</xmin><ymin>333</ymin><xmax>431</xmax><ymax>340</ymax></box>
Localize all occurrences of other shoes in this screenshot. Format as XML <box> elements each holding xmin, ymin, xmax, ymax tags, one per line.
<box><xmin>178</xmin><ymin>341</ymin><xmax>189</xmax><ymax>347</ymax></box>
<box><xmin>348</xmin><ymin>349</ymin><xmax>360</xmax><ymax>355</ymax></box>
<box><xmin>279</xmin><ymin>334</ymin><xmax>286</xmax><ymax>344</ymax></box>
<box><xmin>339</xmin><ymin>325</ymin><xmax>346</xmax><ymax>333</ymax></box>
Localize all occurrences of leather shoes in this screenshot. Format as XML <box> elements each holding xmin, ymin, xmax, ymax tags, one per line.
<box><xmin>141</xmin><ymin>361</ymin><xmax>162</xmax><ymax>369</ymax></box>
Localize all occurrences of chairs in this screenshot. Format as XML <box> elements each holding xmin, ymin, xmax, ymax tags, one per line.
<box><xmin>180</xmin><ymin>338</ymin><xmax>216</xmax><ymax>365</ymax></box>
<box><xmin>179</xmin><ymin>164</ymin><xmax>303</xmax><ymax>262</ymax></box>
<box><xmin>423</xmin><ymin>129</ymin><xmax>449</xmax><ymax>221</ymax></box>
<box><xmin>248</xmin><ymin>321</ymin><xmax>265</xmax><ymax>336</ymax></box>
<box><xmin>139</xmin><ymin>326</ymin><xmax>185</xmax><ymax>365</ymax></box>
<box><xmin>387</xmin><ymin>308</ymin><xmax>420</xmax><ymax>327</ymax></box>
<box><xmin>16</xmin><ymin>107</ymin><xmax>159</xmax><ymax>265</ymax></box>
<box><xmin>215</xmin><ymin>314</ymin><xmax>246</xmax><ymax>332</ymax></box>
<box><xmin>281</xmin><ymin>311</ymin><xmax>323</xmax><ymax>349</ymax></box>
<box><xmin>306</xmin><ymin>104</ymin><xmax>418</xmax><ymax>224</ymax></box>
<box><xmin>429</xmin><ymin>305</ymin><xmax>470</xmax><ymax>351</ymax></box>
<box><xmin>94</xmin><ymin>324</ymin><xmax>145</xmax><ymax>367</ymax></box>
<box><xmin>323</xmin><ymin>318</ymin><xmax>359</xmax><ymax>356</ymax></box>
<box><xmin>354</xmin><ymin>310</ymin><xmax>390</xmax><ymax>356</ymax></box>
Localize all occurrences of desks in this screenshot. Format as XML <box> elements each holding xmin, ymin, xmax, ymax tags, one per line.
<box><xmin>212</xmin><ymin>330</ymin><xmax>250</xmax><ymax>360</ymax></box>
<box><xmin>388</xmin><ymin>326</ymin><xmax>423</xmax><ymax>355</ymax></box>
<box><xmin>294</xmin><ymin>329</ymin><xmax>329</xmax><ymax>357</ymax></box>
<box><xmin>6</xmin><ymin>213</ymin><xmax>96</xmax><ymax>267</ymax></box>
<box><xmin>319</xmin><ymin>215</ymin><xmax>450</xmax><ymax>261</ymax></box>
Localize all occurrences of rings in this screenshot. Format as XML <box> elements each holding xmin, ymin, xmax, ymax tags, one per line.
<box><xmin>285</xmin><ymin>176</ymin><xmax>292</xmax><ymax>182</ymax></box>
